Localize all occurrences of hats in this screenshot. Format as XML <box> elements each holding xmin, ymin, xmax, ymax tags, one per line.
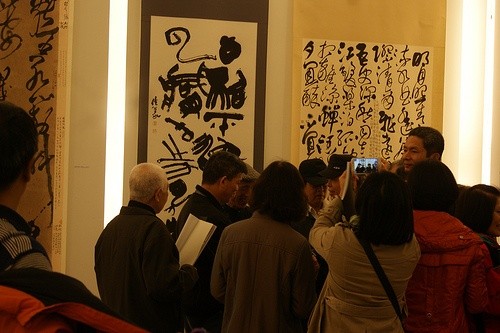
<box><xmin>328</xmin><ymin>154</ymin><xmax>354</xmax><ymax>178</ymax></box>
<box><xmin>299</xmin><ymin>159</ymin><xmax>328</xmax><ymax>185</ymax></box>
<box><xmin>242</xmin><ymin>163</ymin><xmax>261</xmax><ymax>182</ymax></box>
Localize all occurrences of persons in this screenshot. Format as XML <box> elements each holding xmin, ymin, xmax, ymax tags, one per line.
<box><xmin>94</xmin><ymin>162</ymin><xmax>198</xmax><ymax>333</ymax></box>
<box><xmin>172</xmin><ymin>126</ymin><xmax>500</xmax><ymax>333</ymax></box>
<box><xmin>0</xmin><ymin>101</ymin><xmax>53</xmax><ymax>274</ymax></box>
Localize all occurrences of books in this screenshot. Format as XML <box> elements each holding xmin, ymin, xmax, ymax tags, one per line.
<box><xmin>175</xmin><ymin>213</ymin><xmax>218</xmax><ymax>267</ymax></box>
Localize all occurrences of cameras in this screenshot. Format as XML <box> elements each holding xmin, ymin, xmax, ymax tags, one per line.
<box><xmin>351</xmin><ymin>158</ymin><xmax>380</xmax><ymax>174</ymax></box>
